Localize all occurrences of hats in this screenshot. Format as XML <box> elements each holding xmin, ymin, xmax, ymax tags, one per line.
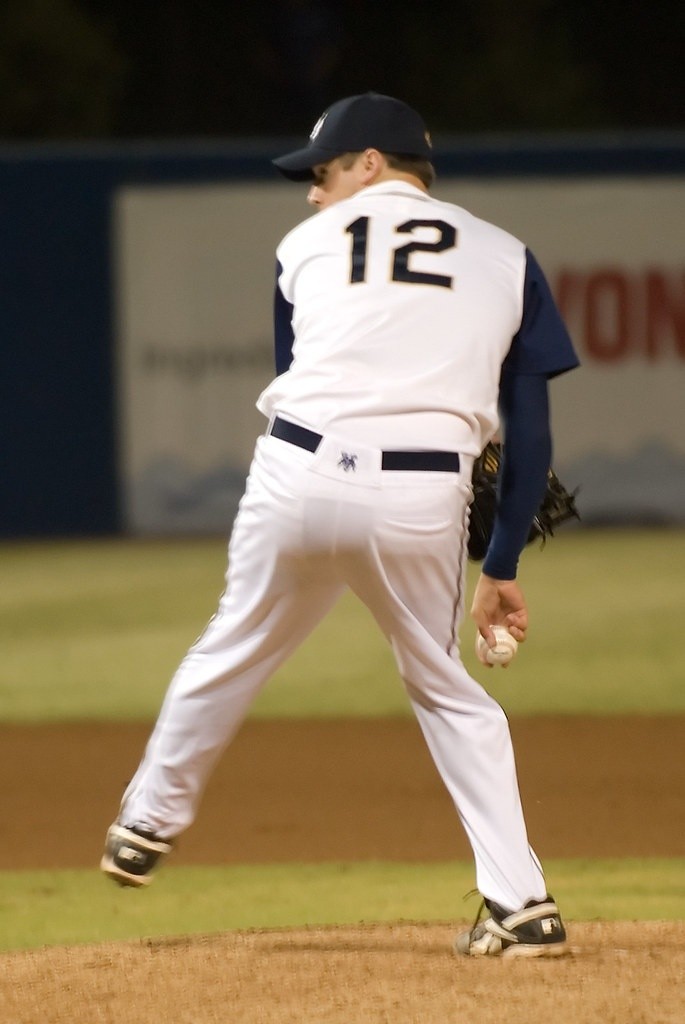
<box><xmin>270</xmin><ymin>91</ymin><xmax>439</xmax><ymax>184</ymax></box>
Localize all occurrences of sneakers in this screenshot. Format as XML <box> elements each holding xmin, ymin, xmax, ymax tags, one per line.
<box><xmin>100</xmin><ymin>819</ymin><xmax>174</xmax><ymax>887</ymax></box>
<box><xmin>454</xmin><ymin>889</ymin><xmax>567</xmax><ymax>959</ymax></box>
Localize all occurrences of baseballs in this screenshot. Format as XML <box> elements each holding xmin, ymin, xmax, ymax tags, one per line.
<box><xmin>474</xmin><ymin>628</ymin><xmax>520</xmax><ymax>664</ymax></box>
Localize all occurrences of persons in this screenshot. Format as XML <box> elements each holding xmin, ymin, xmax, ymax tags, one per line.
<box><xmin>101</xmin><ymin>92</ymin><xmax>571</xmax><ymax>959</ymax></box>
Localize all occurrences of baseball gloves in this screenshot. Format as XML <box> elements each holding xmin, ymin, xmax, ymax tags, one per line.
<box><xmin>470</xmin><ymin>443</ymin><xmax>585</xmax><ymax>565</ymax></box>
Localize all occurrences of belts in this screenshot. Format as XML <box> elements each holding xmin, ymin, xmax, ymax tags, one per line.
<box><xmin>270</xmin><ymin>416</ymin><xmax>461</xmax><ymax>473</ymax></box>
